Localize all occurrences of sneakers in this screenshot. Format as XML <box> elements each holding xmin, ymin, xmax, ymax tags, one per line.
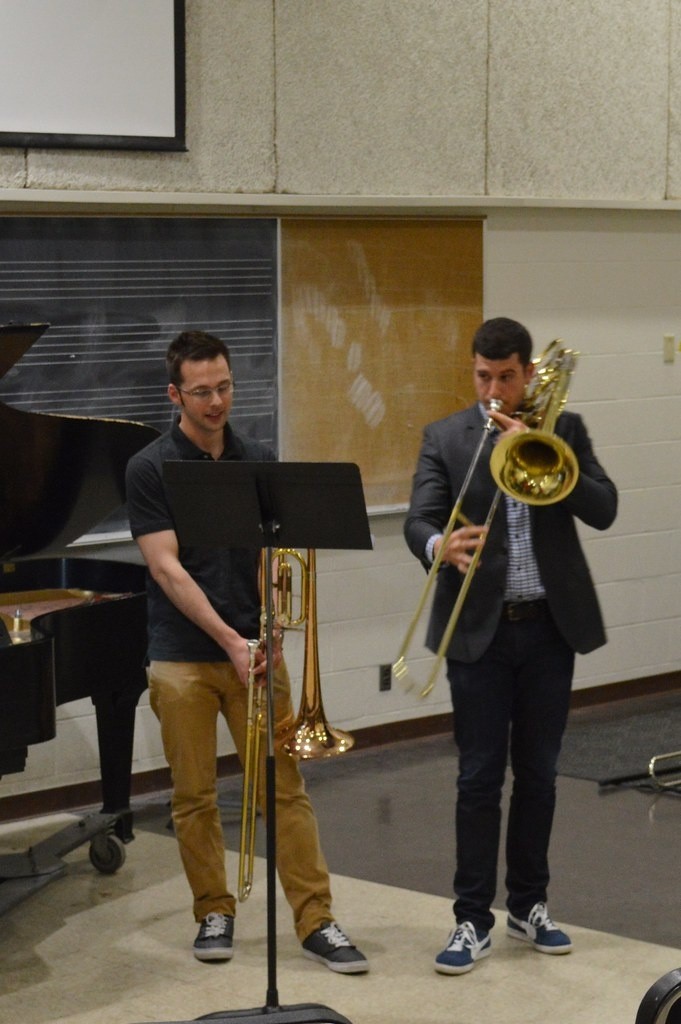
<box><xmin>300</xmin><ymin>922</ymin><xmax>369</xmax><ymax>973</ymax></box>
<box><xmin>506</xmin><ymin>901</ymin><xmax>571</xmax><ymax>954</ymax></box>
<box><xmin>193</xmin><ymin>912</ymin><xmax>235</xmax><ymax>960</ymax></box>
<box><xmin>432</xmin><ymin>916</ymin><xmax>492</xmax><ymax>975</ymax></box>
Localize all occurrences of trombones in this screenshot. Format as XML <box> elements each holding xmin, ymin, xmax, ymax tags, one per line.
<box><xmin>390</xmin><ymin>337</ymin><xmax>579</xmax><ymax>700</ymax></box>
<box><xmin>238</xmin><ymin>544</ymin><xmax>355</xmax><ymax>901</ymax></box>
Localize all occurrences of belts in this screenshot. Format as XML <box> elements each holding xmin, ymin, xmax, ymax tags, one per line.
<box><xmin>501</xmin><ymin>599</ymin><xmax>551</xmax><ymax>625</ymax></box>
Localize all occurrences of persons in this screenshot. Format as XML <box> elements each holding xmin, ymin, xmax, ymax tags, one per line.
<box><xmin>125</xmin><ymin>330</ymin><xmax>370</xmax><ymax>974</ymax></box>
<box><xmin>402</xmin><ymin>317</ymin><xmax>619</xmax><ymax>975</ymax></box>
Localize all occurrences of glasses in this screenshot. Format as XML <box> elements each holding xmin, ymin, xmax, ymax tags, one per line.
<box><xmin>176</xmin><ymin>372</ymin><xmax>232</xmax><ymax>400</ymax></box>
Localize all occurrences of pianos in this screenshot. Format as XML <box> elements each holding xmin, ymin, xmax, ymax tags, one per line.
<box><xmin>0</xmin><ymin>322</ymin><xmax>164</xmax><ymax>844</ymax></box>
